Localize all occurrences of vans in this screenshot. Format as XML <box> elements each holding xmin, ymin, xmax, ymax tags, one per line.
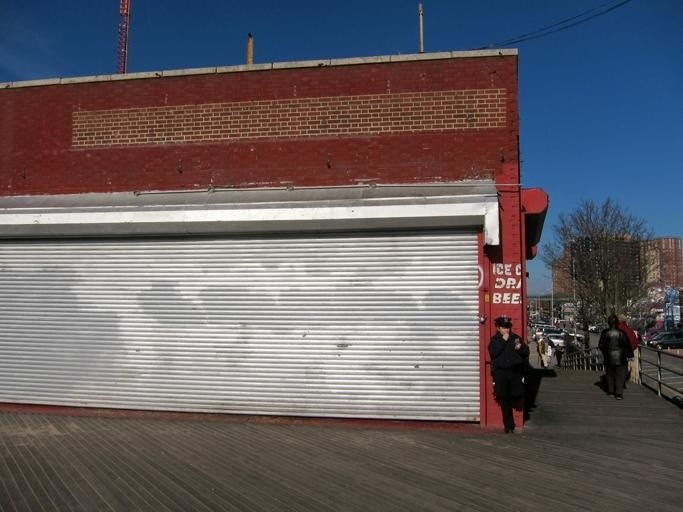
<box><xmin>650</xmin><ymin>332</ymin><xmax>683</xmax><ymax>349</ymax></box>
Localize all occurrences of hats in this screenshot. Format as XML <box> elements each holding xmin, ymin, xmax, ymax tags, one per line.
<box><xmin>494</xmin><ymin>316</ymin><xmax>512</xmax><ymax>327</ymax></box>
<box><xmin>618</xmin><ymin>314</ymin><xmax>627</xmax><ymax>321</ymax></box>
<box><xmin>608</xmin><ymin>314</ymin><xmax>619</xmax><ymax>325</ymax></box>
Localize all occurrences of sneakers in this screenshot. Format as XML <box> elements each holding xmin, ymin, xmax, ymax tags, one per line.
<box><xmin>609</xmin><ymin>392</ymin><xmax>623</xmax><ymax>400</ymax></box>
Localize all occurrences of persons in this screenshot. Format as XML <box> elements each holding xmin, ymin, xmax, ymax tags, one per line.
<box><xmin>553</xmin><ymin>349</ymin><xmax>563</xmax><ymax>369</ymax></box>
<box><xmin>654</xmin><ymin>311</ymin><xmax>665</xmax><ymax>330</ymax></box>
<box><xmin>615</xmin><ymin>314</ymin><xmax>638</xmax><ymax>391</ymax></box>
<box><xmin>486</xmin><ymin>316</ymin><xmax>530</xmax><ymax>433</ymax></box>
<box><xmin>597</xmin><ymin>313</ymin><xmax>635</xmax><ymax>400</ymax></box>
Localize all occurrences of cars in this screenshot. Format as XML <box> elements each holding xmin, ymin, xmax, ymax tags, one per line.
<box><xmin>535</xmin><ymin>327</ymin><xmax>585</xmax><ymax>348</ymax></box>
